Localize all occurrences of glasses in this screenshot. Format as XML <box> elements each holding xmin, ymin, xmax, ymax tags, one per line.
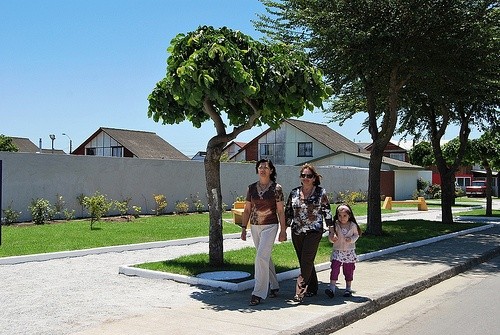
<box><xmin>301</xmin><ymin>173</ymin><xmax>313</xmax><ymax>179</ymax></box>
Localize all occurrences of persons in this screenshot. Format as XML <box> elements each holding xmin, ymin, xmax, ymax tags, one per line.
<box><xmin>285</xmin><ymin>165</ymin><xmax>335</xmax><ymax>304</ymax></box>
<box><xmin>241</xmin><ymin>159</ymin><xmax>286</xmax><ymax>306</ymax></box>
<box><xmin>325</xmin><ymin>203</ymin><xmax>361</xmax><ymax>298</ymax></box>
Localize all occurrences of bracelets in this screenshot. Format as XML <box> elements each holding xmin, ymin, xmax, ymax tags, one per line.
<box><xmin>242</xmin><ymin>225</ymin><xmax>247</xmax><ymax>230</ymax></box>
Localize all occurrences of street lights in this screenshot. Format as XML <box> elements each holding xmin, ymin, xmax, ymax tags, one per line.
<box><xmin>63</xmin><ymin>133</ymin><xmax>73</xmax><ymax>154</ymax></box>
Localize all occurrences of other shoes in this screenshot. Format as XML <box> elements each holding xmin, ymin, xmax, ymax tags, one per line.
<box><xmin>248</xmin><ymin>295</ymin><xmax>262</xmax><ymax>306</ymax></box>
<box><xmin>300</xmin><ymin>277</ymin><xmax>306</xmax><ymax>287</ymax></box>
<box><xmin>268</xmin><ymin>288</ymin><xmax>280</xmax><ymax>298</ymax></box>
<box><xmin>324</xmin><ymin>289</ymin><xmax>335</xmax><ymax>299</ymax></box>
<box><xmin>298</xmin><ymin>286</ymin><xmax>318</xmax><ymax>298</ymax></box>
<box><xmin>343</xmin><ymin>290</ymin><xmax>353</xmax><ymax>297</ymax></box>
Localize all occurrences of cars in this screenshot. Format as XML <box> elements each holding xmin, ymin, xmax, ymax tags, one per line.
<box><xmin>466</xmin><ymin>181</ymin><xmax>494</xmax><ymax>196</ymax></box>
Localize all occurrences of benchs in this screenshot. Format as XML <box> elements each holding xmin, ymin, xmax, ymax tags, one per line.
<box><xmin>382</xmin><ymin>197</ymin><xmax>429</xmax><ymax>211</ymax></box>
<box><xmin>231</xmin><ymin>202</ymin><xmax>251</xmax><ymax>230</ymax></box>
